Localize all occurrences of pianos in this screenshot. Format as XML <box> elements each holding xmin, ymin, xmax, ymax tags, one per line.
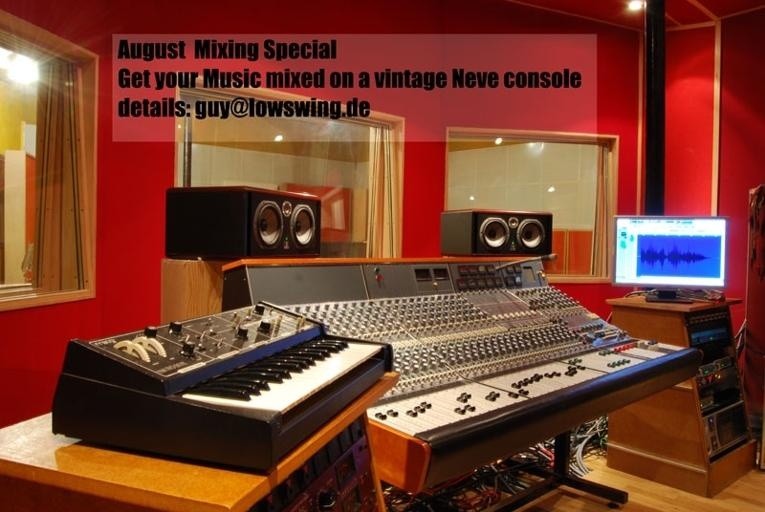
<box><xmin>51</xmin><ymin>301</ymin><xmax>392</xmax><ymax>471</ymax></box>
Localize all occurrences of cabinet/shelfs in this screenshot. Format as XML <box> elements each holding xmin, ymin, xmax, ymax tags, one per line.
<box><xmin>4</xmin><ymin>149</ymin><xmax>35</xmax><ymax>285</ymax></box>
<box><xmin>221</xmin><ymin>255</ymin><xmax>700</xmax><ymax>512</ymax></box>
<box><xmin>158</xmin><ymin>257</ymin><xmax>223</xmax><ymax>326</ymax></box>
<box><xmin>603</xmin><ymin>295</ymin><xmax>758</xmax><ymax>498</ymax></box>
<box><xmin>0</xmin><ymin>373</ymin><xmax>401</xmax><ymax>512</ymax></box>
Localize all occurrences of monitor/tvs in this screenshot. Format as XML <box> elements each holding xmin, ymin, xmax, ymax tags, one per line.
<box><xmin>612</xmin><ymin>215</ymin><xmax>730</xmax><ymax>304</ymax></box>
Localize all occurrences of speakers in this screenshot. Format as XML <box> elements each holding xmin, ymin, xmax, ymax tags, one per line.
<box><xmin>441</xmin><ymin>207</ymin><xmax>553</xmax><ymax>255</ymax></box>
<box><xmin>166</xmin><ymin>185</ymin><xmax>321</xmax><ymax>260</ymax></box>
